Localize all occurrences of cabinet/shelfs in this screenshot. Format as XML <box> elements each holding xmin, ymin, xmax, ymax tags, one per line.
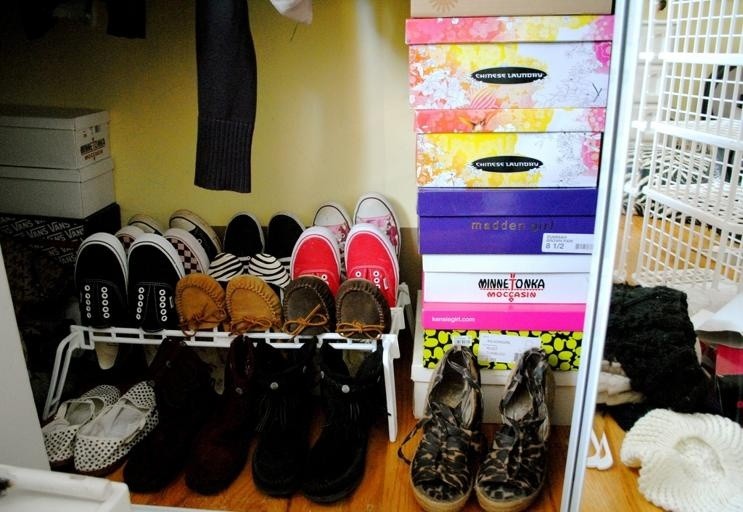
<box><xmin>633</xmin><ymin>1</ymin><xmax>742</xmax><ymax>320</ymax></box>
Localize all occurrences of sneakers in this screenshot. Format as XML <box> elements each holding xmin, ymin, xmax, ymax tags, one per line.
<box><xmin>41</xmin><ymin>382</ymin><xmax>120</xmax><ymax>469</ymax></box>
<box><xmin>114</xmin><ymin>226</ymin><xmax>147</xmax><ymax>260</ymax></box>
<box><xmin>74</xmin><ymin>379</ymin><xmax>161</xmax><ymax>476</ymax></box>
<box><xmin>247</xmin><ymin>254</ymin><xmax>289</xmax><ymax>307</ymax></box>
<box><xmin>169</xmin><ymin>209</ymin><xmax>222</xmax><ymax>261</ymax></box>
<box><xmin>354</xmin><ymin>194</ymin><xmax>401</xmax><ymax>263</ymax></box>
<box><xmin>345</xmin><ymin>223</ymin><xmax>400</xmax><ymax>308</ymax></box>
<box><xmin>264</xmin><ymin>212</ymin><xmax>305</xmax><ymax>258</ymax></box>
<box><xmin>127</xmin><ymin>212</ymin><xmax>167</xmax><ymax>235</ymax></box>
<box><xmin>290</xmin><ymin>226</ymin><xmax>341</xmax><ymax>303</ymax></box>
<box><xmin>224</xmin><ymin>212</ymin><xmax>266</xmax><ymax>259</ymax></box>
<box><xmin>73</xmin><ymin>233</ymin><xmax>130</xmax><ymax>370</ymax></box>
<box><xmin>127</xmin><ymin>233</ymin><xmax>186</xmax><ymax>381</ymax></box>
<box><xmin>476</xmin><ymin>348</ymin><xmax>556</xmax><ymax>512</ymax></box>
<box><xmin>396</xmin><ymin>345</ymin><xmax>484</xmax><ymax>512</ymax></box>
<box><xmin>208</xmin><ymin>254</ymin><xmax>246</xmax><ymax>290</ymax></box>
<box><xmin>312</xmin><ymin>204</ymin><xmax>352</xmax><ymax>279</ymax></box>
<box><xmin>163</xmin><ymin>228</ymin><xmax>208</xmax><ymax>276</ymax></box>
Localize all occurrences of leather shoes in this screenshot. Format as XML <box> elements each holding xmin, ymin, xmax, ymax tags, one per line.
<box><xmin>331</xmin><ymin>277</ymin><xmax>392</xmax><ymax>388</ymax></box>
<box><xmin>225</xmin><ymin>275</ymin><xmax>288</xmax><ymax>393</ymax></box>
<box><xmin>175</xmin><ymin>274</ymin><xmax>234</xmax><ymax>397</ymax></box>
<box><xmin>282</xmin><ymin>274</ymin><xmax>335</xmax><ymax>369</ymax></box>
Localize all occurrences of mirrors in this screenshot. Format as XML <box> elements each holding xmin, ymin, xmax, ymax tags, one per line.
<box><xmin>559</xmin><ymin>0</ymin><xmax>741</xmax><ymax>510</ymax></box>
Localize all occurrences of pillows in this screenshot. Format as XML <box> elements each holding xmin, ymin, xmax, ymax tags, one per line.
<box><xmin>624</xmin><ymin>136</ymin><xmax>718</xmax><ymax>227</ymax></box>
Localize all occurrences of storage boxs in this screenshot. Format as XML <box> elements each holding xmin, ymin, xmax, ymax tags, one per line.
<box><xmin>414</xmin><ymin>190</ymin><xmax>596</xmax><ymax>254</ymax></box>
<box><xmin>1</xmin><ymin>156</ymin><xmax>115</xmax><ymax>220</ymax></box>
<box><xmin>403</xmin><ymin>15</ymin><xmax>614</xmax><ymax>107</ymax></box>
<box><xmin>1</xmin><ymin>205</ymin><xmax>122</xmax><ymax>267</ymax></box>
<box><xmin>411</xmin><ymin>106</ymin><xmax>607</xmax><ymax>189</ymax></box>
<box><xmin>408</xmin><ymin>289</ymin><xmax>578</xmax><ymax>426</ymax></box>
<box><xmin>408</xmin><ymin>2</ymin><xmax>612</xmax><ymax>18</ymax></box>
<box><xmin>421</xmin><ymin>307</ymin><xmax>584</xmax><ymax>372</ymax></box>
<box><xmin>0</xmin><ymin>106</ymin><xmax>111</xmax><ymax>171</ymax></box>
<box><xmin>420</xmin><ymin>254</ymin><xmax>594</xmax><ymax>304</ymax></box>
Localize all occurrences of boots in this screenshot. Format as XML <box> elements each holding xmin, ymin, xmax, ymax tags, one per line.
<box><xmin>302</xmin><ymin>341</ymin><xmax>383</xmax><ymax>503</ymax></box>
<box><xmin>250</xmin><ymin>340</ymin><xmax>314</xmax><ymax>498</ymax></box>
<box><xmin>123</xmin><ymin>341</ymin><xmax>220</xmax><ymax>494</ymax></box>
<box><xmin>185</xmin><ymin>336</ymin><xmax>264</xmax><ymax>496</ymax></box>
